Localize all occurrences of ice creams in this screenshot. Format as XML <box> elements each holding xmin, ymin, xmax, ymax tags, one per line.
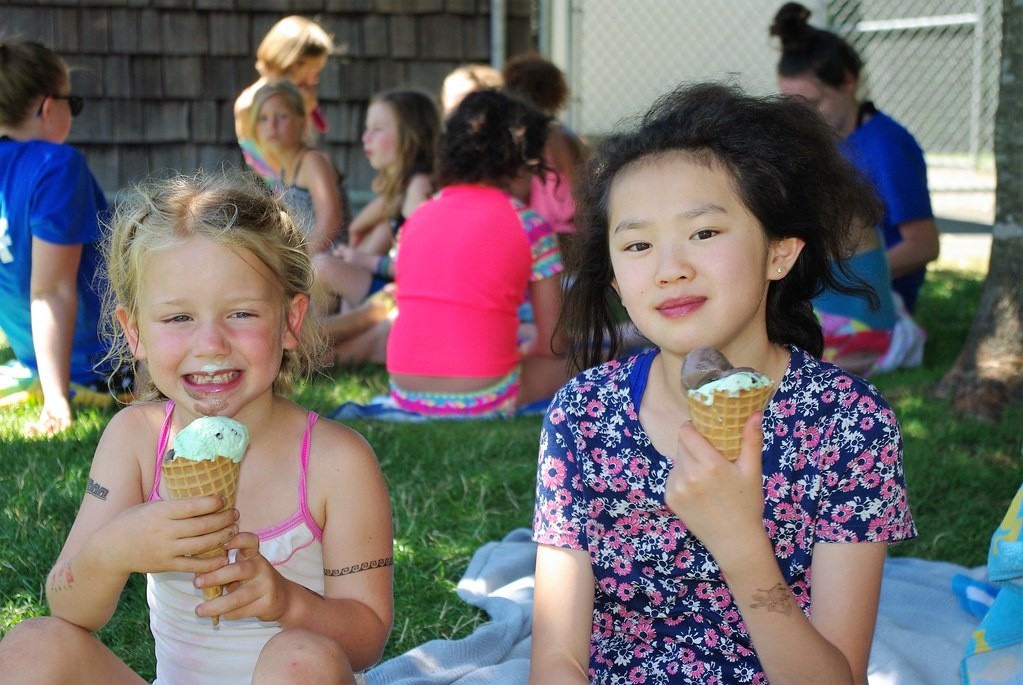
<box><xmin>681</xmin><ymin>344</ymin><xmax>774</xmax><ymax>464</ymax></box>
<box><xmin>161</xmin><ymin>416</ymin><xmax>250</xmax><ymax>625</ymax></box>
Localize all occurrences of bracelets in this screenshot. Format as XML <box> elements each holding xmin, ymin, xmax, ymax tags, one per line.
<box><xmin>377</xmin><ymin>256</ymin><xmax>392</xmax><ymax>277</ymax></box>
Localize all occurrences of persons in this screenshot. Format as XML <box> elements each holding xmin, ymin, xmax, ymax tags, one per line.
<box><xmin>528</xmin><ymin>83</ymin><xmax>920</xmax><ymax>684</ymax></box>
<box><xmin>246</xmin><ymin>80</ymin><xmax>349</xmax><ymax>265</ymax></box>
<box><xmin>232</xmin><ymin>15</ymin><xmax>333</xmax><ymax>196</ymax></box>
<box><xmin>503</xmin><ymin>53</ymin><xmax>593</xmax><ymax>205</ymax></box>
<box><xmin>768</xmin><ymin>3</ymin><xmax>941</xmax><ymax>314</ymax></box>
<box><xmin>0</xmin><ymin>165</ymin><xmax>398</xmax><ymax>685</ymax></box>
<box><xmin>299</xmin><ymin>92</ymin><xmax>442</xmax><ymax>320</ymax></box>
<box><xmin>310</xmin><ymin>88</ymin><xmax>578</xmax><ymax>381</ymax></box>
<box><xmin>809</xmin><ymin>226</ymin><xmax>898</xmax><ymax>384</ymax></box>
<box><xmin>383</xmin><ymin>130</ymin><xmax>580</xmax><ymax>422</ymax></box>
<box><xmin>1</xmin><ymin>33</ymin><xmax>155</xmax><ymax>442</ymax></box>
